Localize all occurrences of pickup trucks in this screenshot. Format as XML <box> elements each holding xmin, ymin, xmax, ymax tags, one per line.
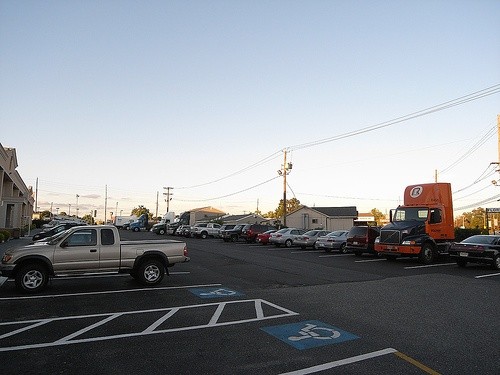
<box><xmin>0</xmin><ymin>225</ymin><xmax>194</xmax><ymax>294</ymax></box>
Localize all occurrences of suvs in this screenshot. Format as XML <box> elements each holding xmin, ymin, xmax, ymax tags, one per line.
<box><xmin>190</xmin><ymin>222</ymin><xmax>274</xmax><ymax>244</ymax></box>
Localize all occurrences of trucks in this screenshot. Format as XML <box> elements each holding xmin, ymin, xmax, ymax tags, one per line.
<box><xmin>373</xmin><ymin>183</ymin><xmax>463</xmax><ymax>265</ymax></box>
<box><xmin>113</xmin><ymin>212</ymin><xmax>195</xmax><ymax>237</ymax></box>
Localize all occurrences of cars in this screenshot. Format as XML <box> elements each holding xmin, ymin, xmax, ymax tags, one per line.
<box><xmin>449</xmin><ymin>234</ymin><xmax>500</xmax><ymax>271</ymax></box>
<box><xmin>292</xmin><ymin>230</ymin><xmax>334</xmax><ymax>250</ymax></box>
<box><xmin>268</xmin><ymin>227</ymin><xmax>306</xmax><ymax>248</ymax></box>
<box><xmin>315</xmin><ymin>230</ymin><xmax>350</xmax><ymax>254</ymax></box>
<box><xmin>255</xmin><ymin>230</ymin><xmax>277</xmax><ymax>246</ymax></box>
<box><xmin>31</xmin><ymin>216</ymin><xmax>87</xmax><ymax>243</ymax></box>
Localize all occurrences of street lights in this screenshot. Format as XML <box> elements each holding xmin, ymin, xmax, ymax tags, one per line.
<box><xmin>76</xmin><ymin>194</ymin><xmax>79</xmax><ymax>216</ymax></box>
<box><xmin>69</xmin><ymin>203</ymin><xmax>71</xmax><ymax>216</ymax></box>
<box><xmin>164</xmin><ymin>197</ymin><xmax>173</xmax><ymax>212</ymax></box>
<box><xmin>56</xmin><ymin>208</ymin><xmax>60</xmax><ymax>216</ymax></box>
<box><xmin>277</xmin><ymin>162</ymin><xmax>293</xmax><ymax>227</ymax></box>
<box><xmin>120</xmin><ymin>210</ymin><xmax>123</xmax><ymax>217</ymax></box>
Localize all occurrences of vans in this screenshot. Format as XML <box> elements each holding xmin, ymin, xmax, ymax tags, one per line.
<box><xmin>346</xmin><ymin>225</ymin><xmax>382</xmax><ymax>256</ymax></box>
